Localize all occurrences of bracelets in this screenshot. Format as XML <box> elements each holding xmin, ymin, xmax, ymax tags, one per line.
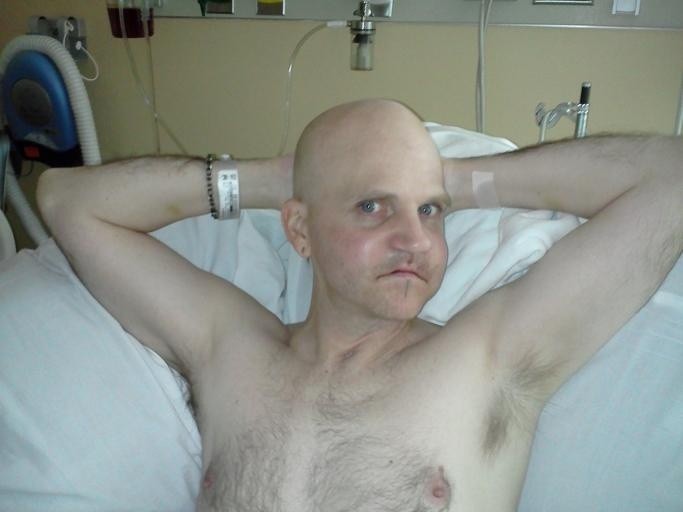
<box><xmin>205</xmin><ymin>153</ymin><xmax>239</xmax><ymax>220</ymax></box>
